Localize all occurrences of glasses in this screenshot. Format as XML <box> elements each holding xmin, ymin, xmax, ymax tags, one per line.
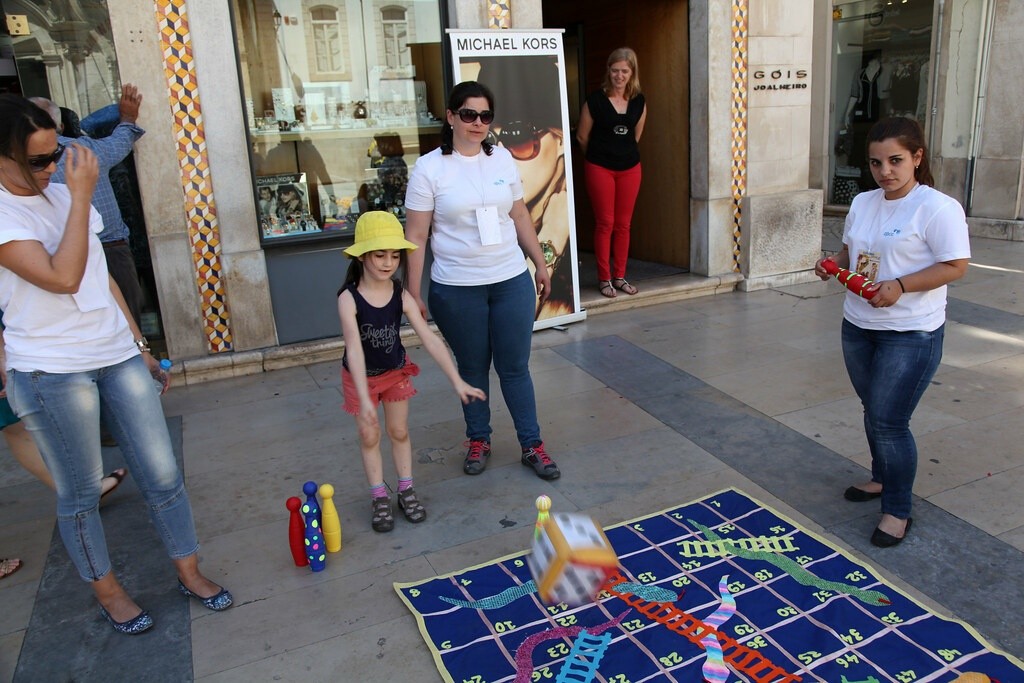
<box><xmin>452</xmin><ymin>109</ymin><xmax>494</xmax><ymax>125</ymax></box>
<box><xmin>486</xmin><ymin>120</ymin><xmax>550</xmax><ymax>160</ymax></box>
<box><xmin>278</xmin><ymin>190</ymin><xmax>293</xmax><ymax>196</ymax></box>
<box><xmin>1</xmin><ymin>142</ymin><xmax>65</xmax><ymax>172</ymax></box>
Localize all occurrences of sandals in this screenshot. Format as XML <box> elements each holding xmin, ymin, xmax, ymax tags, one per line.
<box><xmin>614</xmin><ymin>279</ymin><xmax>638</xmax><ymax>295</ymax></box>
<box><xmin>599</xmin><ymin>280</ymin><xmax>617</xmax><ymax>297</ymax></box>
<box><xmin>0</xmin><ymin>558</ymin><xmax>23</xmax><ymax>579</ymax></box>
<box><xmin>99</xmin><ymin>467</ymin><xmax>127</xmax><ymax>509</ymax></box>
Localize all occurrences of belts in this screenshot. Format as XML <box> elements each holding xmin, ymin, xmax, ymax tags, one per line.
<box><xmin>102</xmin><ymin>239</ymin><xmax>127</xmax><ymax>248</ymax></box>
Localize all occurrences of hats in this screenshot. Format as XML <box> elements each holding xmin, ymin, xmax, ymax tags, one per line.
<box><xmin>342</xmin><ymin>211</ymin><xmax>418</xmax><ymax>259</ymax></box>
<box><xmin>477</xmin><ymin>56</ymin><xmax>561</xmax><ymax>130</ymax></box>
<box><xmin>278</xmin><ymin>182</ymin><xmax>294</xmax><ymax>191</ymax></box>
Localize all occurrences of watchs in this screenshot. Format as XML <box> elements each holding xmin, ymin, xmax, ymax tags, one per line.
<box><xmin>540</xmin><ymin>240</ymin><xmax>562</xmax><ymax>271</ymax></box>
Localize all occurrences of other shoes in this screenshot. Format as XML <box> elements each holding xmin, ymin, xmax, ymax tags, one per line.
<box><xmin>844</xmin><ymin>480</ymin><xmax>882</xmax><ymax>502</ymax></box>
<box><xmin>871</xmin><ymin>516</ymin><xmax>913</xmax><ymax>548</ymax></box>
<box><xmin>177</xmin><ymin>575</ymin><xmax>233</xmax><ymax>611</ymax></box>
<box><xmin>97</xmin><ymin>600</ymin><xmax>153</xmax><ymax>634</ymax></box>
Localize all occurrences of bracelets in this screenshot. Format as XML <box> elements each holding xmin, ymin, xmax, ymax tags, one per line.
<box><xmin>136</xmin><ymin>337</ymin><xmax>148</xmax><ymax>348</ymax></box>
<box><xmin>139</xmin><ymin>348</ymin><xmax>150</xmax><ymax>352</ymax></box>
<box><xmin>896</xmin><ymin>277</ymin><xmax>906</xmax><ymax>293</ymax></box>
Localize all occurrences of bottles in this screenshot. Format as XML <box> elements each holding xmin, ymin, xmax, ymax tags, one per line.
<box><xmin>150</xmin><ymin>358</ymin><xmax>172</xmax><ymax>396</ymax></box>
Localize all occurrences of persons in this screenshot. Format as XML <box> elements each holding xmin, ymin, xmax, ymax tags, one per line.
<box><xmin>0</xmin><ymin>96</ymin><xmax>235</xmax><ymax>636</ymax></box>
<box><xmin>258</xmin><ymin>187</ymin><xmax>278</xmax><ymax>220</ymax></box>
<box><xmin>0</xmin><ymin>305</ymin><xmax>127</xmax><ymax>580</ymax></box>
<box><xmin>276</xmin><ymin>185</ymin><xmax>305</xmax><ymax>217</ymax></box>
<box><xmin>26</xmin><ymin>83</ymin><xmax>146</xmax><ymax>445</ymax></box>
<box><xmin>336</xmin><ymin>211</ymin><xmax>490</xmax><ymax>532</ymax></box>
<box><xmin>403</xmin><ymin>81</ymin><xmax>562</xmax><ymax>482</ymax></box>
<box><xmin>477</xmin><ymin>55</ymin><xmax>574</xmax><ymax>324</ymax></box>
<box><xmin>814</xmin><ymin>118</ymin><xmax>972</xmax><ymax>549</ymax></box>
<box><xmin>373</xmin><ymin>132</ymin><xmax>408</xmax><ymax>192</ymax></box>
<box><xmin>266</xmin><ymin>126</ymin><xmax>338</xmax><ymax>229</ymax></box>
<box><xmin>845</xmin><ymin>59</ymin><xmax>892</xmax><ymax>188</ymax></box>
<box><xmin>576</xmin><ymin>48</ymin><xmax>645</xmax><ymax>298</ymax></box>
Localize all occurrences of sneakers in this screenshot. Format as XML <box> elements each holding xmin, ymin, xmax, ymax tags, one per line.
<box><xmin>463</xmin><ymin>438</ymin><xmax>491</xmax><ymax>475</ymax></box>
<box><xmin>522</xmin><ymin>441</ymin><xmax>560</xmax><ymax>480</ymax></box>
<box><xmin>398</xmin><ymin>487</ymin><xmax>426</xmax><ymax>523</ymax></box>
<box><xmin>372</xmin><ymin>496</ymin><xmax>394</xmax><ymax>532</ymax></box>
